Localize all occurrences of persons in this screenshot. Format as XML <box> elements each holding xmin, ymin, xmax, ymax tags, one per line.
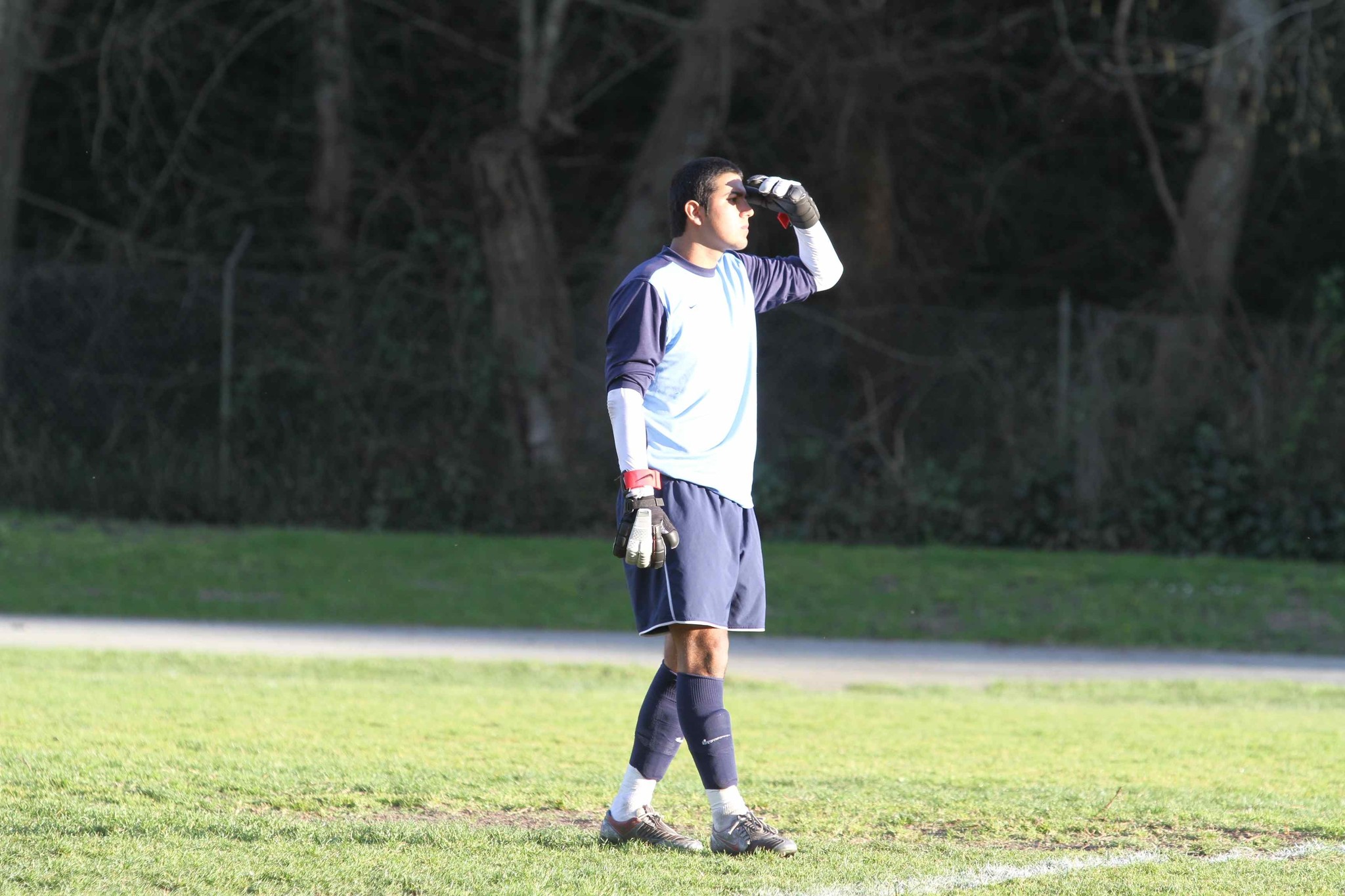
<box><xmin>598</xmin><ymin>157</ymin><xmax>845</xmax><ymax>861</ymax></box>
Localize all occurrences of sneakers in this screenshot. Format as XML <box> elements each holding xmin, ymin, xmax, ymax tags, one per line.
<box><xmin>597</xmin><ymin>804</ymin><xmax>703</xmax><ymax>854</ymax></box>
<box><xmin>709</xmin><ymin>812</ymin><xmax>798</xmax><ymax>856</ymax></box>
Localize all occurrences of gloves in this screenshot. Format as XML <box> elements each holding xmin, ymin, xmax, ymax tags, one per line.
<box><xmin>744</xmin><ymin>173</ymin><xmax>820</xmax><ymax>229</ymax></box>
<box><xmin>613</xmin><ymin>469</ymin><xmax>680</xmax><ymax>569</ymax></box>
